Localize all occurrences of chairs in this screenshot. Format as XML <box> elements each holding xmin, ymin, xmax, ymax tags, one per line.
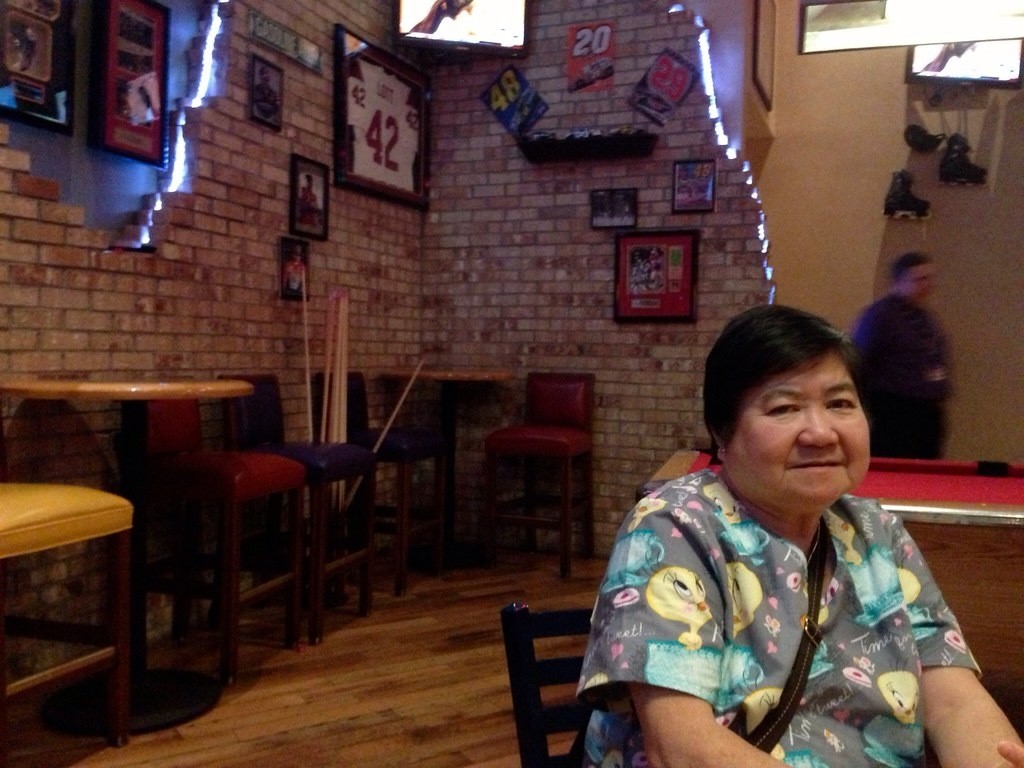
<box><xmin>502</xmin><ymin>601</ymin><xmax>594</xmax><ymax>768</ymax></box>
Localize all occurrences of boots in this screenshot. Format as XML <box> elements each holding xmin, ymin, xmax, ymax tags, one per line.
<box><xmin>939</xmin><ymin>133</ymin><xmax>987</xmax><ymax>185</ymax></box>
<box><xmin>884</xmin><ymin>169</ymin><xmax>931</xmax><ymax>214</ymax></box>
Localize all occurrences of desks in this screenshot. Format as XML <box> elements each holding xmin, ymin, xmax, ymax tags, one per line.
<box><xmin>0</xmin><ymin>378</ymin><xmax>254</xmax><ymax>737</ymax></box>
<box><xmin>380</xmin><ymin>366</ymin><xmax>501</xmax><ymax>575</ymax></box>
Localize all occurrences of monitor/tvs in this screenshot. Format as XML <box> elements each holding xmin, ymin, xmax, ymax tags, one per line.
<box><xmin>903</xmin><ymin>38</ymin><xmax>1024</xmax><ymax>89</ymax></box>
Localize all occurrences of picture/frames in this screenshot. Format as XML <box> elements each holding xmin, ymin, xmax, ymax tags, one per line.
<box><xmin>90</xmin><ymin>3</ymin><xmax>171</xmax><ymax>169</ymax></box>
<box><xmin>0</xmin><ymin>0</ymin><xmax>79</xmax><ymax>136</ymax></box>
<box><xmin>672</xmin><ymin>159</ymin><xmax>716</xmax><ymax>213</ymax></box>
<box><xmin>612</xmin><ymin>228</ymin><xmax>696</xmax><ymax>323</ymax></box>
<box><xmin>333</xmin><ymin>19</ymin><xmax>430</xmax><ymax>205</ymax></box>
<box><xmin>247</xmin><ymin>51</ymin><xmax>284</xmax><ymax>129</ymax></box>
<box><xmin>289</xmin><ymin>152</ymin><xmax>330</xmax><ymax>241</ymax></box>
<box><xmin>278</xmin><ymin>234</ymin><xmax>310</xmax><ymax>301</ymax></box>
<box><xmin>590</xmin><ymin>187</ymin><xmax>636</xmax><ymax>229</ymax></box>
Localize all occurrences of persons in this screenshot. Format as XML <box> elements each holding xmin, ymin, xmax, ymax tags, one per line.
<box><xmin>574</xmin><ymin>305</ymin><xmax>1024</xmax><ymax>768</ymax></box>
<box><xmin>850</xmin><ymin>253</ymin><xmax>950</xmax><ymax>460</ymax></box>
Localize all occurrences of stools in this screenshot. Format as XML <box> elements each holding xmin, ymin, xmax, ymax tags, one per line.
<box><xmin>481</xmin><ymin>373</ymin><xmax>598</xmax><ymax>583</ymax></box>
<box><xmin>0</xmin><ymin>481</ymin><xmax>130</xmax><ymax>753</ymax></box>
<box><xmin>132</xmin><ymin>370</ymin><xmax>434</xmax><ymax>690</ymax></box>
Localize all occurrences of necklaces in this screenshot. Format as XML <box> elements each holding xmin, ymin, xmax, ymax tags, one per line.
<box><xmin>807</xmin><ymin>521</ymin><xmax>820</xmax><ymax>564</ymax></box>
